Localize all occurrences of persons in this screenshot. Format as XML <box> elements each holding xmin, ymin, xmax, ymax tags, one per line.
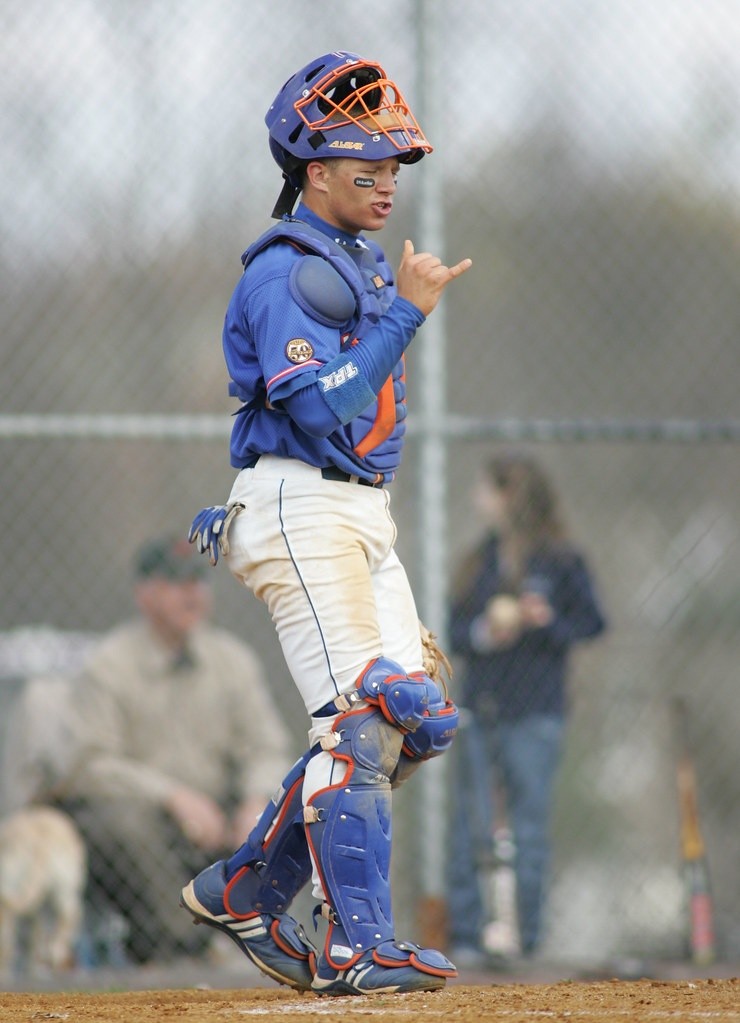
<box><xmin>181</xmin><ymin>49</ymin><xmax>474</xmax><ymax>1000</ymax></box>
<box><xmin>440</xmin><ymin>448</ymin><xmax>608</xmax><ymax>971</ymax></box>
<box><xmin>43</xmin><ymin>528</ymin><xmax>301</xmax><ymax>974</ymax></box>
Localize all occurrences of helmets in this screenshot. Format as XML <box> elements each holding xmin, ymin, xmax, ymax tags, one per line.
<box><xmin>266</xmin><ymin>51</ymin><xmax>433</xmax><ymax>187</ymax></box>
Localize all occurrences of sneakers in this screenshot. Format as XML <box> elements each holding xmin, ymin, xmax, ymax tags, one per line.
<box><xmin>311</xmin><ymin>950</ymin><xmax>445</xmax><ymax>993</ymax></box>
<box><xmin>184</xmin><ymin>862</ymin><xmax>314</xmax><ymax>993</ymax></box>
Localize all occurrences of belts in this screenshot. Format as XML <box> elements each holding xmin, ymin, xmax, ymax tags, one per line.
<box><xmin>243</xmin><ymin>455</ymin><xmax>382</xmax><ymax>491</ymax></box>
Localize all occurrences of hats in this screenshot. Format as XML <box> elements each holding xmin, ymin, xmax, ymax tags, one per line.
<box><xmin>130</xmin><ymin>526</ymin><xmax>215</xmax><ymax>584</ymax></box>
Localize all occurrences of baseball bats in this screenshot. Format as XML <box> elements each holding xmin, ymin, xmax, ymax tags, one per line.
<box><xmin>660</xmin><ymin>687</ymin><xmax>724</xmax><ymax>970</ymax></box>
